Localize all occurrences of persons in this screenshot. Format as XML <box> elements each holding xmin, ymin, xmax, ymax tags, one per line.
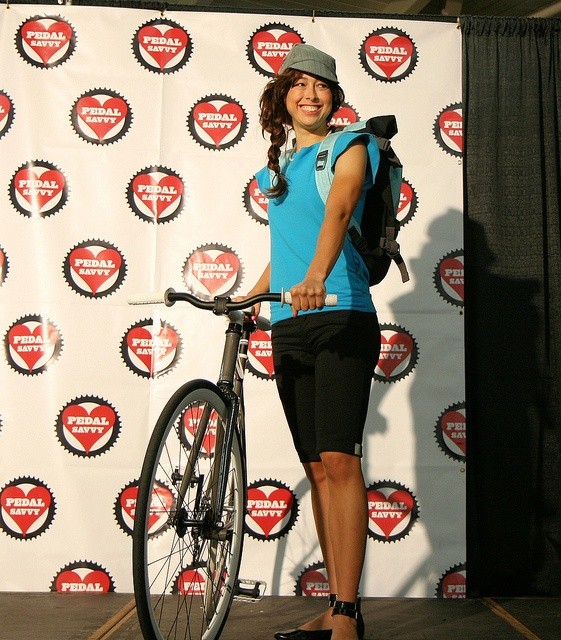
<box><xmin>232</xmin><ymin>44</ymin><xmax>382</xmax><ymax>639</ymax></box>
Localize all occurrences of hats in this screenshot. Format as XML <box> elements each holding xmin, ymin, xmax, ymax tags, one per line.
<box><xmin>278</xmin><ymin>44</ymin><xmax>340</xmax><ymax>83</ymax></box>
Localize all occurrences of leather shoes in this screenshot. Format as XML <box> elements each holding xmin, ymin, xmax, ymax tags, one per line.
<box><xmin>331</xmin><ymin>597</ymin><xmax>365</xmax><ymax>639</ymax></box>
<box><xmin>274</xmin><ymin>593</ymin><xmax>336</xmax><ymax>640</ymax></box>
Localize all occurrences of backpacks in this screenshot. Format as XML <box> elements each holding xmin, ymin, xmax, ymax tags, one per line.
<box><xmin>269</xmin><ymin>115</ymin><xmax>409</xmax><ymax>287</ymax></box>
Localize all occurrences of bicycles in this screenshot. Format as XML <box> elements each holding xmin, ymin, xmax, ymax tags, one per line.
<box><xmin>126</xmin><ymin>287</ymin><xmax>338</xmax><ymax>639</ymax></box>
